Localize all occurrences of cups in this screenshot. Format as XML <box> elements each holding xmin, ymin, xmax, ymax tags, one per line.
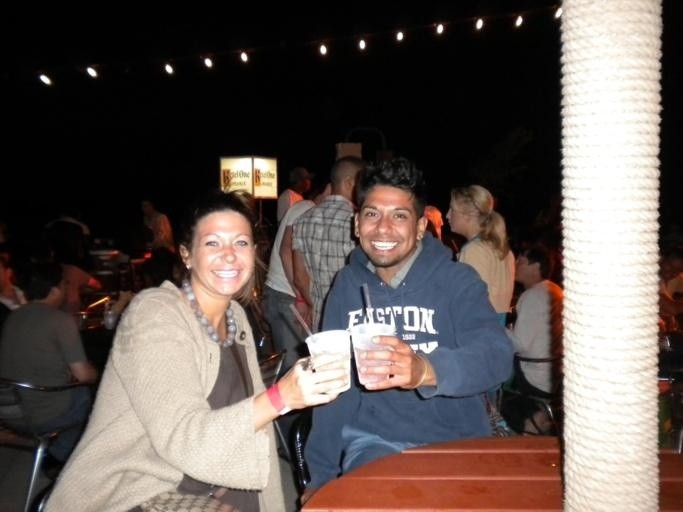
<box><xmin>351</xmin><ymin>323</ymin><xmax>396</xmax><ymax>385</ymax></box>
<box><xmin>305</xmin><ymin>331</ymin><xmax>351</xmax><ymax>393</ymax></box>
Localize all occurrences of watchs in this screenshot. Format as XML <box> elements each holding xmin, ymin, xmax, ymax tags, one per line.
<box><xmin>268</xmin><ymin>384</ymin><xmax>290</xmax><ymax>417</ymax></box>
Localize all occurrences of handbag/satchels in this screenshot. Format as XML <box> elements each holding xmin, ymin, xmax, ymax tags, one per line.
<box><xmin>140</xmin><ymin>490</ymin><xmax>244</xmax><ymax>512</ymax></box>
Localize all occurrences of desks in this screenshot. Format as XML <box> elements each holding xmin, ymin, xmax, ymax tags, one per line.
<box><xmin>83</xmin><ymin>319</ymin><xmax>118</xmax><ymax>367</ymax></box>
<box><xmin>295</xmin><ymin>434</ymin><xmax>683</xmax><ymax>512</ymax></box>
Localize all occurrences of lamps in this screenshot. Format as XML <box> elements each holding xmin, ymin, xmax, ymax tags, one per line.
<box><xmin>218</xmin><ymin>144</ymin><xmax>281</xmax><ymax>200</ymax></box>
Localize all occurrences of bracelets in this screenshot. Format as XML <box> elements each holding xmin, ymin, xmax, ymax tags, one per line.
<box><xmin>295</xmin><ymin>298</ymin><xmax>305</xmax><ymax>303</ymax></box>
<box><xmin>405</xmin><ymin>352</ymin><xmax>428</xmax><ymax>389</ymax></box>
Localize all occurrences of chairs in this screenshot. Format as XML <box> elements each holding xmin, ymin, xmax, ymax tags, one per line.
<box><xmin>253</xmin><ymin>345</ymin><xmax>296</xmax><ymax>466</ymax></box>
<box><xmin>506</xmin><ymin>352</ymin><xmax>564</xmax><ymax>429</ymax></box>
<box><xmin>655</xmin><ymin>333</ymin><xmax>683</xmax><ymax>431</ymax></box>
<box><xmin>0</xmin><ymin>377</ymin><xmax>92</xmax><ymax>512</ymax></box>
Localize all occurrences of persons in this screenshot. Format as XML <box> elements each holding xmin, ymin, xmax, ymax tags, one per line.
<box><xmin>238</xmin><ymin>192</ymin><xmax>275</xmax><ymax>355</ymax></box>
<box><xmin>277</xmin><ymin>170</ymin><xmax>310</xmax><ymax>232</ymax></box>
<box><xmin>64</xmin><ymin>265</ymin><xmax>102</xmax><ymax>312</ymax></box>
<box><xmin>500</xmin><ymin>246</ymin><xmax>561</xmax><ymax>434</ymax></box>
<box><xmin>300</xmin><ymin>156</ymin><xmax>515</xmax><ymax>503</ymax></box>
<box><xmin>447</xmin><ymin>186</ymin><xmax>514</xmax><ymax>437</ymax></box>
<box><xmin>261</xmin><ymin>166</ymin><xmax>333</xmax><ymax>452</ymax></box>
<box><xmin>40</xmin><ymin>191</ymin><xmax>347</xmax><ymax>512</ymax></box>
<box><xmin>0</xmin><ymin>254</ymin><xmax>26</xmax><ymax>317</ymax></box>
<box><xmin>138</xmin><ymin>198</ymin><xmax>182</xmax><ymax>287</ymax></box>
<box><xmin>293</xmin><ymin>159</ymin><xmax>358</xmax><ymax>338</ymax></box>
<box><xmin>3</xmin><ymin>254</ymin><xmax>97</xmax><ymax>476</ymax></box>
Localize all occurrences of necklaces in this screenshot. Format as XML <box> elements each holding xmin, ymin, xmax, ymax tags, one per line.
<box><xmin>181</xmin><ymin>275</ymin><xmax>236</xmax><ymax>347</ymax></box>
